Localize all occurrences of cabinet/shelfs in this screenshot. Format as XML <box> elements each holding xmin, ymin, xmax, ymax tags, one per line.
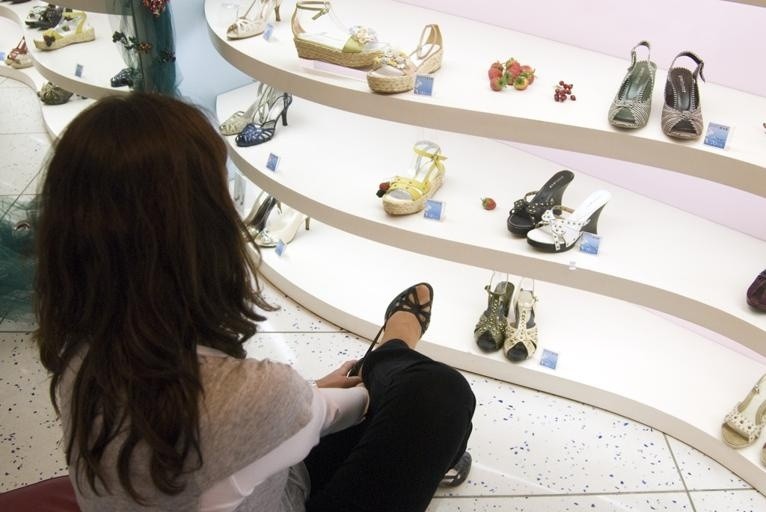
<box><xmin>0</xmin><ymin>0</ymin><xmax>138</xmax><ymax>150</ymax></box>
<box><xmin>202</xmin><ymin>0</ymin><xmax>766</xmax><ymax>498</ymax></box>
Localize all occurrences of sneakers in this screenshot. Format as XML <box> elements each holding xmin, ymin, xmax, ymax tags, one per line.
<box><xmin>746</xmin><ymin>267</ymin><xmax>766</xmax><ymax>316</ymax></box>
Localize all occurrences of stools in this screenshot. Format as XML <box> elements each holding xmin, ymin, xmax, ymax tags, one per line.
<box><xmin>0</xmin><ymin>474</ymin><xmax>82</xmax><ymax>512</ymax></box>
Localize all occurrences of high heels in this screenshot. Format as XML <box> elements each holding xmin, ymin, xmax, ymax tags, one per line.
<box><xmin>218</xmin><ymin>81</ymin><xmax>293</xmax><ymax>148</ymax></box>
<box><xmin>506</xmin><ymin>168</ymin><xmax>577</xmax><ymax>241</ymax></box>
<box><xmin>524</xmin><ymin>188</ymin><xmax>613</xmax><ymax>255</ymax></box>
<box><xmin>237</xmin><ymin>188</ymin><xmax>312</xmax><ymax>248</ymax></box>
<box><xmin>226</xmin><ymin>0</ymin><xmax>282</xmax><ymax>40</ymax></box>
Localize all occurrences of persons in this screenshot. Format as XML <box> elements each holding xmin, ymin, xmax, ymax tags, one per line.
<box><xmin>29</xmin><ymin>92</ymin><xmax>477</xmax><ymax>512</ymax></box>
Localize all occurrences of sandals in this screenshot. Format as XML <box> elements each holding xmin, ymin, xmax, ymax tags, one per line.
<box><xmin>719</xmin><ymin>371</ymin><xmax>766</xmax><ymax>469</ymax></box>
<box><xmin>366</xmin><ymin>23</ymin><xmax>444</xmax><ymax>94</ymax></box>
<box><xmin>4</xmin><ymin>2</ymin><xmax>143</xmax><ymax>109</ymax></box>
<box><xmin>437</xmin><ymin>450</ymin><xmax>473</xmax><ymax>490</ymax></box>
<box><xmin>660</xmin><ymin>48</ymin><xmax>706</xmax><ymax>142</ymax></box>
<box><xmin>343</xmin><ymin>280</ymin><xmax>435</xmax><ymax>387</ymax></box>
<box><xmin>500</xmin><ymin>274</ymin><xmax>542</xmax><ymax>363</ymax></box>
<box><xmin>607</xmin><ymin>38</ymin><xmax>658</xmax><ymax>131</ymax></box>
<box><xmin>472</xmin><ymin>270</ymin><xmax>515</xmax><ymax>354</ymax></box>
<box><xmin>380</xmin><ymin>138</ymin><xmax>447</xmax><ymax>216</ymax></box>
<box><xmin>291</xmin><ymin>0</ymin><xmax>385</xmax><ymax>69</ymax></box>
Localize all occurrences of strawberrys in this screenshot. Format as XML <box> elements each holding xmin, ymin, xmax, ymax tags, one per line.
<box><xmin>489</xmin><ymin>57</ymin><xmax>535</xmax><ymax>91</ymax></box>
<box><xmin>379</xmin><ymin>183</ymin><xmax>389</xmax><ymax>192</ymax></box>
<box><xmin>481</xmin><ymin>197</ymin><xmax>496</xmax><ymax>210</ymax></box>
